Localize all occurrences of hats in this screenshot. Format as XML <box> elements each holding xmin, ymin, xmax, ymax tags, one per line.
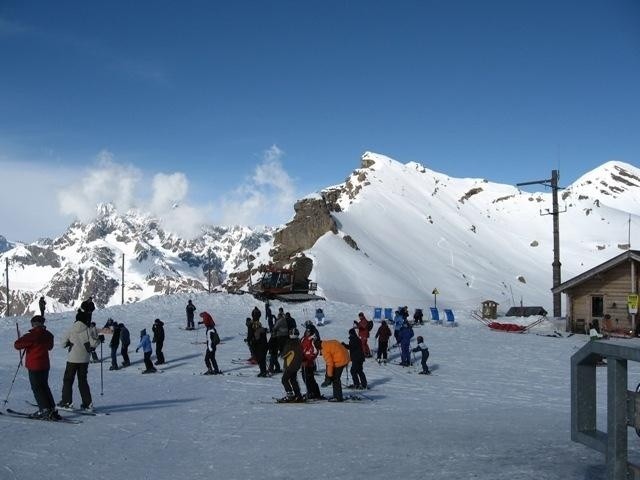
<box><xmin>31</xmin><ymin>315</ymin><xmax>45</xmax><ymax>323</ymax></box>
<box><xmin>76</xmin><ymin>309</ymin><xmax>89</xmax><ymax>324</ymax></box>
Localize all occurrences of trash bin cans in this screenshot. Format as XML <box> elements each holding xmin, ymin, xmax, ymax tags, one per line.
<box><xmin>481</xmin><ymin>300</ymin><xmax>499</xmax><ymax>319</ymax></box>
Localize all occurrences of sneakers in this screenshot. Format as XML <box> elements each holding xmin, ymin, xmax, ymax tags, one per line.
<box><xmin>29</xmin><ymin>407</ymin><xmax>54</xmax><ymax>418</ymax></box>
<box><xmin>321</xmin><ymin>377</ymin><xmax>332</xmax><ymax>387</ymax></box>
<box><xmin>56</xmin><ymin>400</ymin><xmax>72</xmax><ymax>408</ymax></box>
<box><xmin>81</xmin><ymin>402</ymin><xmax>91</xmax><ymax>410</ymax></box>
<box><xmin>281</xmin><ymin>391</ymin><xmax>343</xmax><ymax>402</ymax></box>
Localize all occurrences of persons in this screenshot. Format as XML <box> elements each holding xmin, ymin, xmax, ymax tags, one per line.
<box><xmin>244</xmin><ymin>301</ymin><xmax>429</xmax><ymax>402</ymax></box>
<box><xmin>152</xmin><ymin>318</ymin><xmax>166</xmax><ymax>365</ymax></box>
<box><xmin>135</xmin><ymin>328</ymin><xmax>154</xmax><ymax>371</ymax></box>
<box><xmin>185</xmin><ymin>300</ymin><xmax>197</xmax><ymax>328</ymax></box>
<box><xmin>13</xmin><ymin>315</ymin><xmax>58</xmax><ymax>420</ymax></box>
<box><xmin>197</xmin><ymin>311</ymin><xmax>216</xmax><ymax>334</ymax></box>
<box><xmin>57</xmin><ymin>312</ymin><xmax>102</xmax><ymax>408</ymax></box>
<box><xmin>38</xmin><ymin>296</ymin><xmax>46</xmax><ymax>317</ymax></box>
<box><xmin>81</xmin><ymin>296</ymin><xmax>131</xmax><ymax>371</ymax></box>
<box><xmin>205</xmin><ymin>324</ymin><xmax>219</xmax><ymax>375</ymax></box>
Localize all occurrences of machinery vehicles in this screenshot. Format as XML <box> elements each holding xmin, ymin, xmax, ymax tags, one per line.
<box><xmin>249</xmin><ymin>256</ymin><xmax>327</xmax><ymax>303</ymax></box>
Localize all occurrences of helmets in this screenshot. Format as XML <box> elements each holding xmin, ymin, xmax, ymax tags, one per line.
<box><xmin>288</xmin><ymin>328</ymin><xmax>299</xmax><ymax>339</ymax></box>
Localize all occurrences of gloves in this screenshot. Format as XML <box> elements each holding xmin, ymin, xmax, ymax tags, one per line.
<box><xmin>99</xmin><ymin>334</ymin><xmax>104</xmax><ymax>343</ymax></box>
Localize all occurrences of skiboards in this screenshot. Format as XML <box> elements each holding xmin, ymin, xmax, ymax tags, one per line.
<box><xmin>191</xmin><ymin>341</ymin><xmax>226</xmax><ymax>345</ymax></box>
<box><xmin>407</xmin><ymin>371</ymin><xmax>439</xmax><ymax>376</ymax></box>
<box><xmin>192</xmin><ymin>371</ymin><xmax>243</xmax><ymax>377</ymax></box>
<box><xmin>536</xmin><ymin>330</ymin><xmax>564</xmax><ymax>338</ymax></box>
<box><xmin>230</xmin><ymin>358</ymin><xmax>272</xmax><ymax>365</ymax></box>
<box><xmin>370</xmin><ymin>343</ymin><xmax>415</xmax><ymax>367</ymax></box>
<box><xmin>250</xmin><ymin>397</ymin><xmax>326</xmax><ymax>404</ymax></box>
<box><xmin>179</xmin><ymin>326</ymin><xmax>205</xmax><ymax>330</ymax></box>
<box><xmin>341</xmin><ymin>385</ymin><xmax>378</xmax><ymax>390</ymax></box>
<box><xmin>26</xmin><ymin>400</ymin><xmax>111</xmax><ymax>416</ymax></box>
<box><xmin>323</xmin><ymin>395</ymin><xmax>372</xmax><ymax>403</ymax></box>
<box><xmin>300</xmin><ymin>368</ymin><xmax>326</xmax><ymax>376</ymax></box>
<box><xmin>0</xmin><ymin>409</ymin><xmax>83</xmax><ymax>425</ymax></box>
